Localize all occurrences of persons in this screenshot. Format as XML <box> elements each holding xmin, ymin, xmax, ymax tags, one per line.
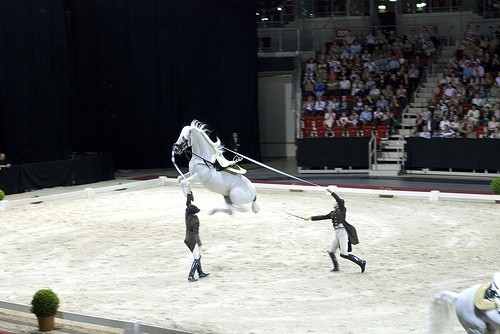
<box><xmin>413</xmin><ymin>25</ymin><xmax>500</xmax><ymax>139</ymax></box>
<box><xmin>227</xmin><ymin>133</ymin><xmax>241</xmax><ymax>159</ymax></box>
<box><xmin>304</xmin><ymin>187</ymin><xmax>366</xmax><ymax>274</ymax></box>
<box><xmin>299</xmin><ymin>24</ymin><xmax>437</xmax><ymax>130</ymax></box>
<box><xmin>0</xmin><ymin>154</ymin><xmax>11</xmax><ymax>170</ymax></box>
<box><xmin>183</xmin><ymin>191</ymin><xmax>210</xmax><ymax>282</ymax></box>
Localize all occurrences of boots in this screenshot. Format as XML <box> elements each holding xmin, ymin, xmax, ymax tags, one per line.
<box><xmin>188</xmin><ymin>259</ymin><xmax>200</xmax><ymax>281</ymax></box>
<box><xmin>340</xmin><ymin>253</ymin><xmax>366</xmax><ymax>273</ymax></box>
<box><xmin>328</xmin><ymin>251</ymin><xmax>339</xmax><ymax>271</ymax></box>
<box><xmin>196</xmin><ymin>262</ymin><xmax>209</xmax><ymax>277</ymax></box>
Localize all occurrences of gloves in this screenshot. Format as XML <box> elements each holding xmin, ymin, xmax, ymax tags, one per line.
<box><xmin>304</xmin><ymin>216</ymin><xmax>311</xmax><ymax>221</ymax></box>
<box><xmin>200</xmin><ymin>245</ymin><xmax>207</xmax><ymax>251</ymax></box>
<box><xmin>326</xmin><ymin>188</ymin><xmax>334</xmax><ymax>194</ymax></box>
<box><xmin>187</xmin><ymin>185</ymin><xmax>191</xmax><ymax>194</ymax></box>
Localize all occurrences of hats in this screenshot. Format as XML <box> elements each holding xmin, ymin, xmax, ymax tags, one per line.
<box><xmin>186</xmin><ymin>205</ymin><xmax>200</xmax><ymax>214</ymax></box>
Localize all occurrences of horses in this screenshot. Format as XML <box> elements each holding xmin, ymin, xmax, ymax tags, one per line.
<box><xmin>171</xmin><ymin>118</ymin><xmax>261</xmax><ymax>217</ymax></box>
<box><xmin>424</xmin><ymin>269</ymin><xmax>500</xmax><ymax>334</ymax></box>
